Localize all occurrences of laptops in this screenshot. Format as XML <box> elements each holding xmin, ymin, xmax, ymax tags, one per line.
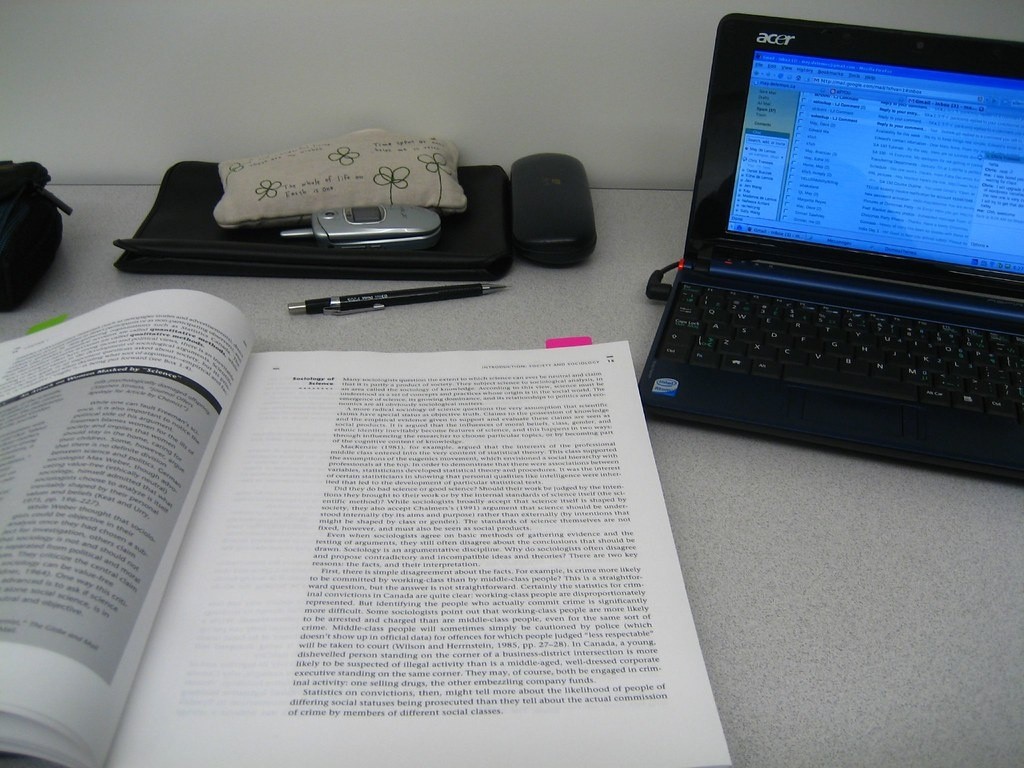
<box><xmin>638</xmin><ymin>12</ymin><xmax>1024</xmax><ymax>487</ymax></box>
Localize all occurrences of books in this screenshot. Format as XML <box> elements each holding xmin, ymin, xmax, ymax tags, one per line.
<box><xmin>0</xmin><ymin>287</ymin><xmax>736</xmax><ymax>768</ymax></box>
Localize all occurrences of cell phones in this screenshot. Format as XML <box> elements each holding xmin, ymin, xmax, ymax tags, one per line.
<box><xmin>280</xmin><ymin>205</ymin><xmax>442</xmax><ymax>252</ymax></box>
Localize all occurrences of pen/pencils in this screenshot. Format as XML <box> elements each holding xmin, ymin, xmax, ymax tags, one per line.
<box><xmin>287</xmin><ymin>283</ymin><xmax>512</xmax><ymax>316</ymax></box>
